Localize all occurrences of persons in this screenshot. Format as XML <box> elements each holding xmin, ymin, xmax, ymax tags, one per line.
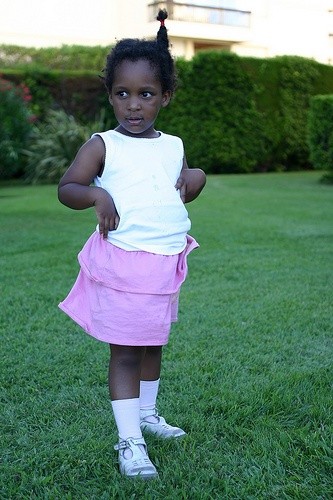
<box><xmin>54</xmin><ymin>9</ymin><xmax>206</xmax><ymax>479</ymax></box>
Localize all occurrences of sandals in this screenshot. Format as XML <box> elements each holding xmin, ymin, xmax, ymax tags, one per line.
<box><xmin>137</xmin><ymin>408</ymin><xmax>186</xmax><ymax>441</ymax></box>
<box><xmin>115</xmin><ymin>436</ymin><xmax>158</xmax><ymax>478</ymax></box>
